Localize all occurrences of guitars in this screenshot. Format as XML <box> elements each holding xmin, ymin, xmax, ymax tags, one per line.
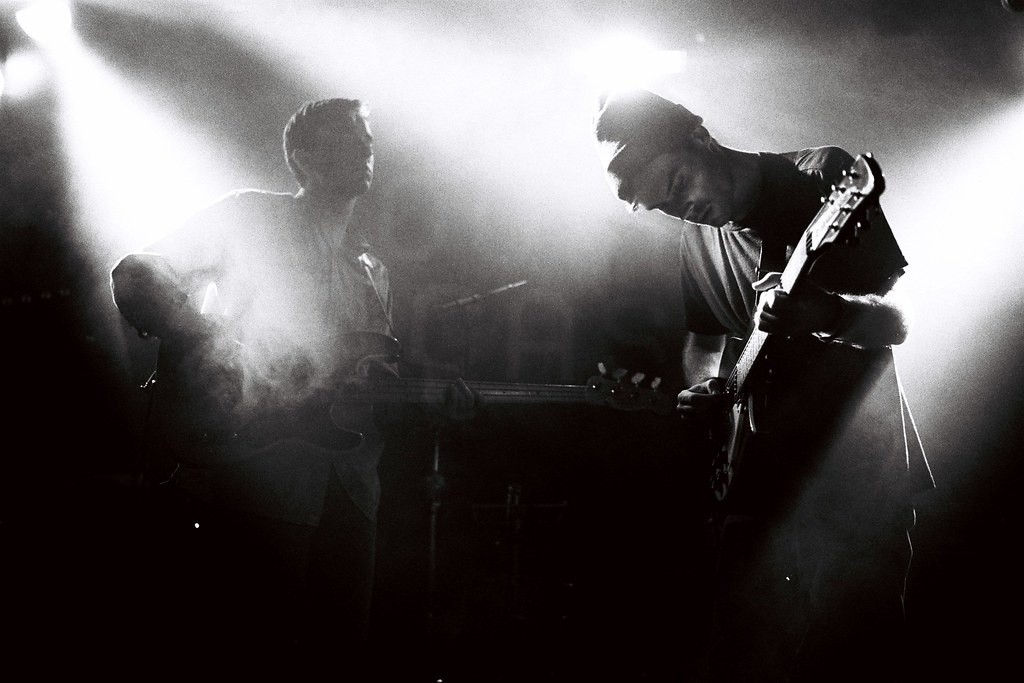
<box><xmin>703</xmin><ymin>153</ymin><xmax>885</xmax><ymax>521</ymax></box>
<box><xmin>145</xmin><ymin>330</ymin><xmax>672</xmax><ymax>473</ymax></box>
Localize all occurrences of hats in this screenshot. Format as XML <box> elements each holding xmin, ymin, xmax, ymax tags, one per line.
<box><xmin>594</xmin><ymin>86</ymin><xmax>703</xmax><ymax>203</ymax></box>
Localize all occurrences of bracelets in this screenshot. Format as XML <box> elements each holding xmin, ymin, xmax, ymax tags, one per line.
<box><xmin>814</xmin><ymin>291</ymin><xmax>847</xmax><ymax>342</ymax></box>
<box><xmin>137</xmin><ymin>289</ymin><xmax>191</xmax><ymax>340</ymax></box>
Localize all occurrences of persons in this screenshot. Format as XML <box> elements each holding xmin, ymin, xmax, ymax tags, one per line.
<box><xmin>594</xmin><ymin>83</ymin><xmax>937</xmax><ymax>683</ymax></box>
<box><xmin>108</xmin><ymin>98</ymin><xmax>477</xmax><ymax>683</ymax></box>
